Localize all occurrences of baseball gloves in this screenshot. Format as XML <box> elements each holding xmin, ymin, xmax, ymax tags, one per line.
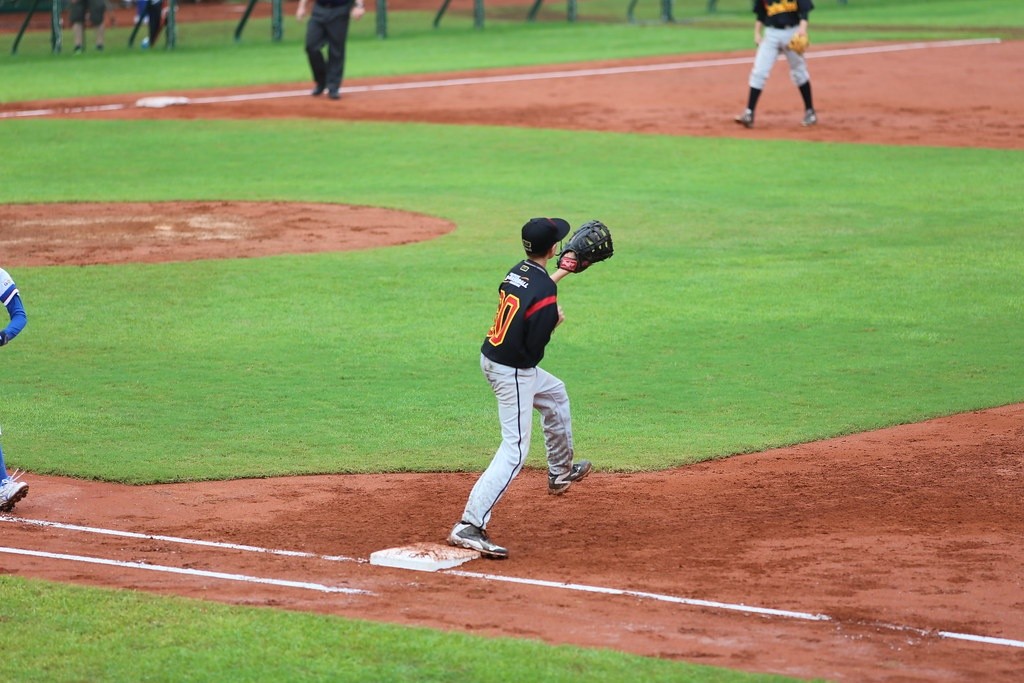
<box><xmin>555</xmin><ymin>220</ymin><xmax>615</xmax><ymax>274</ymax></box>
<box><xmin>786</xmin><ymin>33</ymin><xmax>812</xmax><ymax>52</ymax></box>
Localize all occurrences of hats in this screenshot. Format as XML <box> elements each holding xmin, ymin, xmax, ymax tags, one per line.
<box><xmin>522</xmin><ymin>218</ymin><xmax>570</xmax><ymax>251</ymax></box>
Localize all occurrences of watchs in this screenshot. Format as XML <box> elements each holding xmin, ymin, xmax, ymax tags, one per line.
<box><xmin>354</xmin><ymin>2</ymin><xmax>363</xmax><ymax>7</ymax></box>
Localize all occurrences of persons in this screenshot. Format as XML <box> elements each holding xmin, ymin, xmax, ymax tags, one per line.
<box><xmin>733</xmin><ymin>0</ymin><xmax>817</xmax><ymax>130</ymax></box>
<box><xmin>0</xmin><ymin>267</ymin><xmax>29</xmax><ymax>514</ymax></box>
<box><xmin>69</xmin><ymin>0</ymin><xmax>107</xmax><ymax>54</ymax></box>
<box><xmin>296</xmin><ymin>0</ymin><xmax>365</xmax><ymax>100</ymax></box>
<box><xmin>446</xmin><ymin>218</ymin><xmax>614</xmax><ymax>559</ymax></box>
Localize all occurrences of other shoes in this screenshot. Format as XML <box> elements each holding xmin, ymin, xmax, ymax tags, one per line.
<box><xmin>329</xmin><ymin>88</ymin><xmax>338</xmax><ymax>98</ymax></box>
<box><xmin>312</xmin><ymin>82</ymin><xmax>325</xmax><ymax>94</ymax></box>
<box><xmin>735</xmin><ymin>108</ymin><xmax>754</xmax><ymax>128</ymax></box>
<box><xmin>801</xmin><ymin>109</ymin><xmax>816</xmax><ymax>126</ymax></box>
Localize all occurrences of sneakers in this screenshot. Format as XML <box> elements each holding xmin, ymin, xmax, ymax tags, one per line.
<box><xmin>447</xmin><ymin>522</ymin><xmax>508</xmax><ymax>558</ymax></box>
<box><xmin>548</xmin><ymin>461</ymin><xmax>592</xmax><ymax>495</ymax></box>
<box><xmin>0</xmin><ymin>468</ymin><xmax>29</xmax><ymax>512</ymax></box>
